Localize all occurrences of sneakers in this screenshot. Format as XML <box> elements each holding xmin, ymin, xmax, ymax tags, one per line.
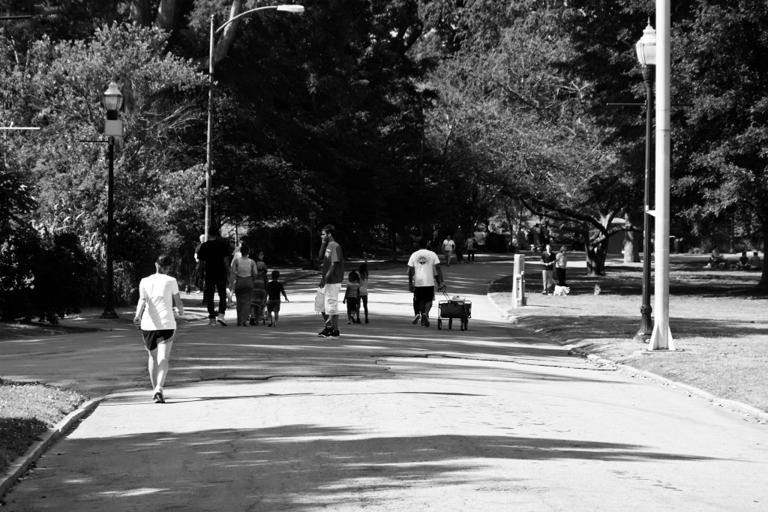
<box><xmin>152</xmin><ymin>387</ymin><xmax>165</xmax><ymax>403</ymax></box>
<box><xmin>318</xmin><ymin>327</ymin><xmax>339</xmax><ymax>340</ymax></box>
<box><xmin>347</xmin><ymin>314</ymin><xmax>368</xmax><ymax>324</ymax></box>
<box><xmin>412</xmin><ymin>313</ymin><xmax>430</xmax><ymax>326</ymax></box>
<box><xmin>207</xmin><ymin>315</ymin><xmax>279</xmax><ymax>327</ymax></box>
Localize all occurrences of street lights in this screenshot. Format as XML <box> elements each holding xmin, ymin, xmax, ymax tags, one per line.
<box><xmin>631</xmin><ymin>15</ymin><xmax>656</xmax><ymax>344</ymax></box>
<box><xmin>101</xmin><ymin>82</ymin><xmax>124</xmax><ymax>317</ymax></box>
<box><xmin>202</xmin><ymin>2</ymin><xmax>305</xmax><ymax>250</ymax></box>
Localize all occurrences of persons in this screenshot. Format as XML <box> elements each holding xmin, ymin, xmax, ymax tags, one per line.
<box><xmin>408</xmin><ymin>239</ymin><xmax>441</xmax><ymax>327</ymax></box>
<box><xmin>180</xmin><ymin>233</ymin><xmax>289</xmax><ymax>327</ymax></box>
<box><xmin>319</xmin><ymin>224</ymin><xmax>344</xmax><ymax>337</ymax></box>
<box><xmin>750</xmin><ymin>252</ymin><xmax>761</xmax><ymax>270</ymax></box>
<box><xmin>201</xmin><ymin>226</ymin><xmax>230</xmax><ymax>326</ymax></box>
<box><xmin>132</xmin><ymin>254</ymin><xmax>184</xmax><ymax>403</ymax></box>
<box><xmin>541</xmin><ymin>244</ymin><xmax>556</xmax><ymax>294</ymax></box>
<box><xmin>556</xmin><ymin>246</ymin><xmax>567</xmax><ymax>286</ymax></box>
<box><xmin>343</xmin><ymin>270</ymin><xmax>361</xmax><ymax>324</ymax></box>
<box><xmin>710</xmin><ymin>246</ymin><xmax>724</xmax><ymax>264</ymax></box>
<box><xmin>736</xmin><ymin>251</ymin><xmax>749</xmax><ymax>269</ymax></box>
<box><xmin>442</xmin><ymin>226</ymin><xmax>477</xmax><ymax>266</ymax></box>
<box><xmin>230</xmin><ymin>247</ymin><xmax>257</xmax><ymax>326</ymax></box>
<box><xmin>357</xmin><ymin>262</ymin><xmax>368</xmax><ymax>324</ymax></box>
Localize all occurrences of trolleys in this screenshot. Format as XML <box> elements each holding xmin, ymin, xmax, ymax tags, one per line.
<box><xmin>438</xmin><ymin>285</ymin><xmax>472</xmax><ymax>331</ymax></box>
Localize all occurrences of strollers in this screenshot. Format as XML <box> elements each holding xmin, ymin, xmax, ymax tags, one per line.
<box><xmin>249</xmin><ymin>288</ymin><xmax>267</xmax><ymax>325</ymax></box>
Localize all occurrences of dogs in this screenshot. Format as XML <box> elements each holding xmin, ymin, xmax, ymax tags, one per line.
<box><xmin>554</xmin><ymin>284</ymin><xmax>570</xmax><ymax>296</ymax></box>
